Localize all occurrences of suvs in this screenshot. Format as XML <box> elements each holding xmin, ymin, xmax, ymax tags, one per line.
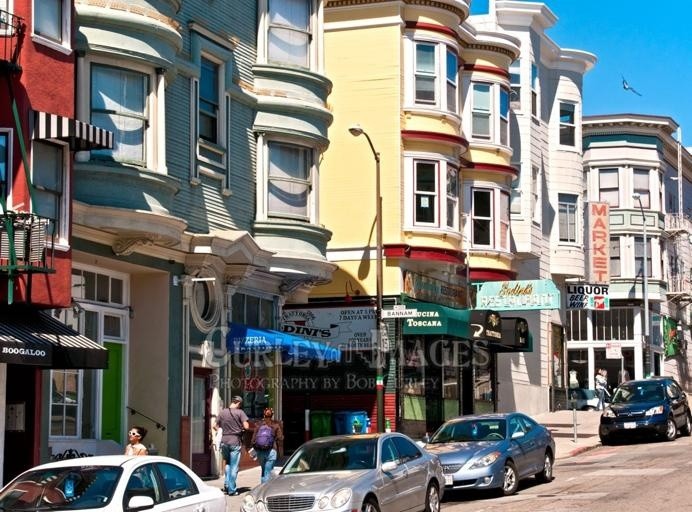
<box><xmin>599</xmin><ymin>376</ymin><xmax>692</xmax><ymax>446</ymax></box>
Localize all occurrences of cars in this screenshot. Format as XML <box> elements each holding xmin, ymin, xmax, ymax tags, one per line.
<box><xmin>422</xmin><ymin>413</ymin><xmax>555</xmax><ymax>496</ymax></box>
<box><xmin>240</xmin><ymin>433</ymin><xmax>445</xmax><ymax>512</ymax></box>
<box><xmin>1</xmin><ymin>455</ymin><xmax>227</xmax><ymax>512</ymax></box>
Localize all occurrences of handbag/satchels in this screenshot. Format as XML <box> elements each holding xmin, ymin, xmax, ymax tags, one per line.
<box><xmin>241</xmin><ymin>430</ymin><xmax>248</xmax><ymax>443</ymax></box>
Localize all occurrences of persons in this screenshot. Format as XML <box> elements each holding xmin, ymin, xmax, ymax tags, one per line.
<box><xmin>124</xmin><ymin>426</ymin><xmax>149</xmax><ymax>482</ymax></box>
<box><xmin>213</xmin><ymin>395</ymin><xmax>249</xmax><ymax>496</ymax></box>
<box><xmin>251</xmin><ymin>406</ymin><xmax>284</xmax><ymax>485</ymax></box>
<box><xmin>595</xmin><ymin>368</ymin><xmax>608</xmax><ymax>411</ymax></box>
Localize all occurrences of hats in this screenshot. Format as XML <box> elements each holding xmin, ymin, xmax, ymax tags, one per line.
<box><xmin>232</xmin><ymin>395</ymin><xmax>243</xmax><ymax>409</ymax></box>
<box><xmin>264</xmin><ymin>408</ymin><xmax>273</xmax><ymax>416</ymax></box>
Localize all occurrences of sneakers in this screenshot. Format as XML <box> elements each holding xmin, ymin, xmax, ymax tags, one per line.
<box><xmin>229</xmin><ymin>491</ymin><xmax>239</xmax><ymax>496</ymax></box>
<box><xmin>223</xmin><ymin>485</ymin><xmax>228</xmax><ymax>491</ymax></box>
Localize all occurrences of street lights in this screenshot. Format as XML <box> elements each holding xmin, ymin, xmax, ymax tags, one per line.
<box><xmin>632</xmin><ymin>193</ymin><xmax>651</xmax><ymax>379</ymax></box>
<box><xmin>351</xmin><ymin>126</ymin><xmax>384</xmax><ymax>433</ymax></box>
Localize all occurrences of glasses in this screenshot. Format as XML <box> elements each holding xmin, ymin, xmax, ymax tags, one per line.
<box><xmin>129</xmin><ymin>431</ymin><xmax>140</xmax><ymax>437</ymax></box>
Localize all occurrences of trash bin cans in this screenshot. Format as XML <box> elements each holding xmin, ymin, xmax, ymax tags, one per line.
<box><xmin>334</xmin><ymin>409</ymin><xmax>370</xmax><ymax>435</ymax></box>
<box><xmin>310</xmin><ymin>411</ymin><xmax>331</xmax><ymax>439</ymax></box>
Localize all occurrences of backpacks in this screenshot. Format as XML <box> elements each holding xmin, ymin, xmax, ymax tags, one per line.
<box><xmin>253</xmin><ymin>424</ymin><xmax>275</xmax><ymax>450</ymax></box>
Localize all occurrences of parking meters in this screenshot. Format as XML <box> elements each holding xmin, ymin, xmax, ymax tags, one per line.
<box><xmin>570</xmin><ymin>391</ymin><xmax>577</xmax><ymax>441</ymax></box>
